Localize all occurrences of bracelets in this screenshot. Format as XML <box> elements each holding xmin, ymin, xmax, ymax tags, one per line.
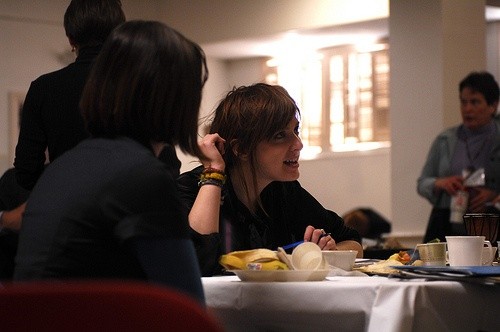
<box><xmin>198</xmin><ymin>167</ymin><xmax>228</xmax><ymax>189</ymax></box>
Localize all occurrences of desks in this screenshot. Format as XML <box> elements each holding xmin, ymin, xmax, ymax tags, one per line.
<box><xmin>202</xmin><ymin>276</ymin><xmax>500</xmax><ymax>332</ymax></box>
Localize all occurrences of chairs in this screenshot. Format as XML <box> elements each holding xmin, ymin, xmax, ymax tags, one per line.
<box><xmin>0</xmin><ymin>281</ymin><xmax>221</xmax><ymax>332</ymax></box>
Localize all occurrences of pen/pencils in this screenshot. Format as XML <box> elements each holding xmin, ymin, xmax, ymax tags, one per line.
<box><xmin>283</xmin><ymin>232</ymin><xmax>332</xmax><ymax>250</ymax></box>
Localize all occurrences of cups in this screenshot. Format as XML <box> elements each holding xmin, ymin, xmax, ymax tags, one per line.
<box><xmin>450</xmin><ymin>190</ymin><xmax>469</xmax><ymax>223</ymax></box>
<box><xmin>291</xmin><ymin>242</ymin><xmax>324</xmax><ymax>270</ymax></box>
<box><xmin>446</xmin><ymin>236</ymin><xmax>492</xmax><ymax>266</ymax></box>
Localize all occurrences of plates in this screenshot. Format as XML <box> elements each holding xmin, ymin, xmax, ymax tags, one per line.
<box><xmin>309</xmin><ymin>271</ymin><xmax>329</xmax><ymax>281</ymax></box>
<box><xmin>226</xmin><ymin>269</ymin><xmax>315</xmax><ymax>282</ymax></box>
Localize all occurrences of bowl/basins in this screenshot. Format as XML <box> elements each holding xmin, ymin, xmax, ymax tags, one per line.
<box><xmin>482</xmin><ymin>247</ymin><xmax>497</xmax><ymax>265</ymax></box>
<box><xmin>417</xmin><ymin>243</ymin><xmax>448</xmax><ymax>267</ymax></box>
<box><xmin>321</xmin><ymin>250</ymin><xmax>359</xmax><ymax>272</ymax></box>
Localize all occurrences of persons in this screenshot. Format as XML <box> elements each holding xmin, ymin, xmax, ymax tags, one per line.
<box><xmin>176</xmin><ymin>82</ymin><xmax>364</xmax><ymax>277</ymax></box>
<box><xmin>0</xmin><ymin>0</ymin><xmax>182</xmax><ymax>215</ymax></box>
<box><xmin>11</xmin><ymin>19</ymin><xmax>207</xmax><ymax>311</ymax></box>
<box><xmin>416</xmin><ymin>71</ymin><xmax>500</xmax><ymax>243</ymax></box>
